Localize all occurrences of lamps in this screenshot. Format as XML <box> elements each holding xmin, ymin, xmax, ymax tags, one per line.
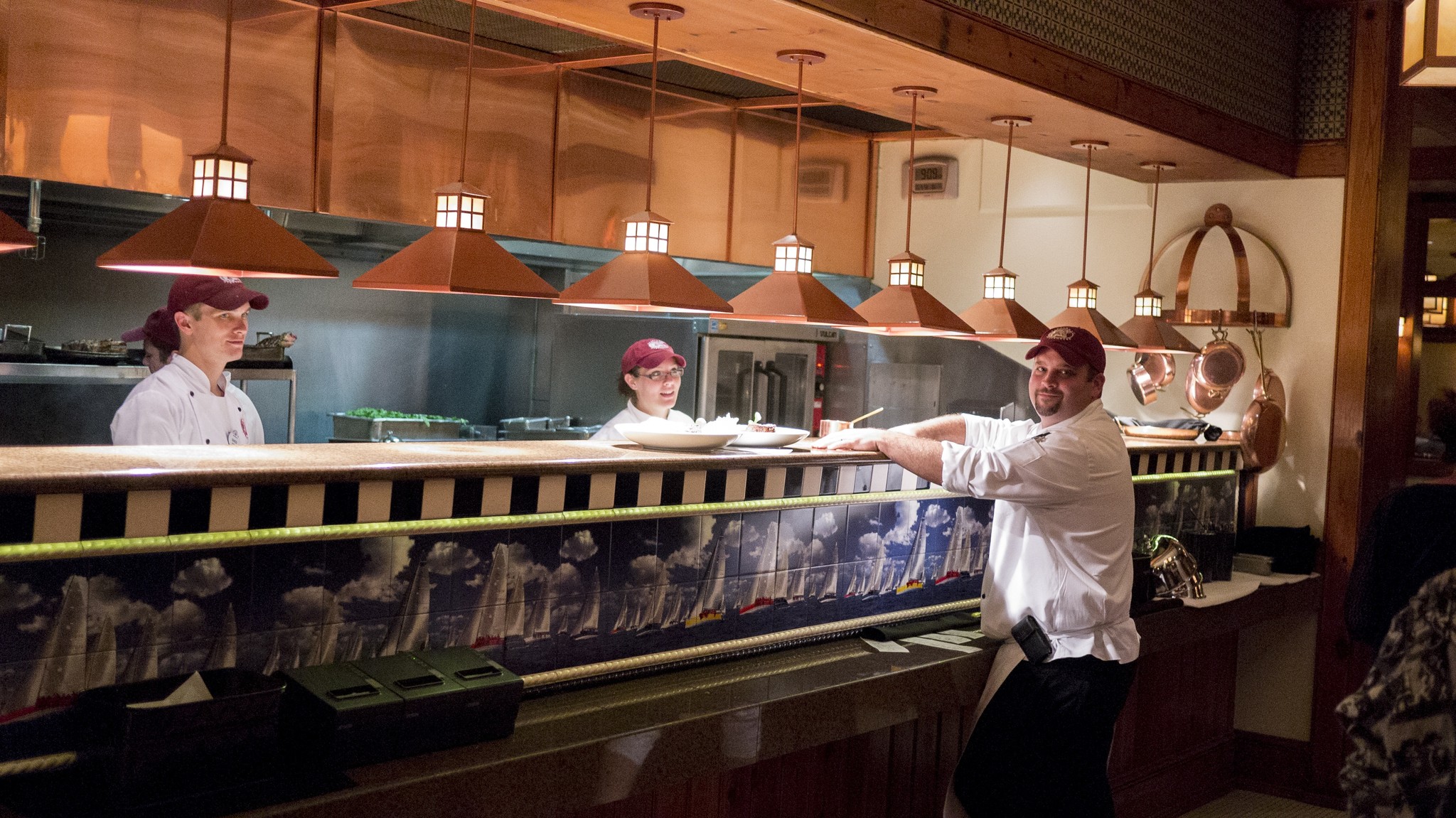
<box><xmin>351</xmin><ymin>0</ymin><xmax>563</xmax><ymax>299</ymax></box>
<box><xmin>1116</xmin><ymin>160</ymin><xmax>1200</xmax><ymax>355</ymax></box>
<box><xmin>1397</xmin><ymin>306</ymin><xmax>1408</xmax><ymax>337</ymax></box>
<box><xmin>1399</xmin><ymin>0</ymin><xmax>1456</xmax><ymax>87</ymax></box>
<box><xmin>1043</xmin><ymin>139</ymin><xmax>1140</xmax><ymax>349</ymax></box>
<box><xmin>96</xmin><ymin>1</ymin><xmax>340</xmax><ymax>283</ymax></box>
<box><xmin>843</xmin><ymin>85</ymin><xmax>976</xmax><ymax>336</ymax></box>
<box><xmin>552</xmin><ymin>2</ymin><xmax>734</xmax><ymax>313</ymax></box>
<box><xmin>957</xmin><ymin>116</ymin><xmax>1052</xmax><ymax>343</ymax></box>
<box><xmin>711</xmin><ymin>48</ymin><xmax>869</xmax><ymax>328</ymax></box>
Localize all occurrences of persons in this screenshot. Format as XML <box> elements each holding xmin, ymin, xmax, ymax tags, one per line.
<box><xmin>118</xmin><ymin>305</ymin><xmax>184</xmax><ymax>377</ymax></box>
<box><xmin>1347</xmin><ymin>389</ymin><xmax>1456</xmax><ymax>649</ymax></box>
<box><xmin>811</xmin><ymin>325</ymin><xmax>1143</xmax><ymax>818</ymax></box>
<box><xmin>109</xmin><ymin>268</ymin><xmax>266</xmax><ymax>448</ymax></box>
<box><xmin>587</xmin><ymin>337</ymin><xmax>697</xmax><ymax>447</ymax></box>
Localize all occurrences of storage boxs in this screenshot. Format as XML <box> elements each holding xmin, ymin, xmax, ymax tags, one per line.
<box><xmin>405</xmin><ymin>644</ymin><xmax>524</xmax><ymax>743</ymax></box>
<box><xmin>347</xmin><ymin>654</ymin><xmax>467</xmax><ymax>756</ymax></box>
<box><xmin>70</xmin><ymin>662</ymin><xmax>295</xmax><ymax>818</ymax></box>
<box><xmin>274</xmin><ymin>662</ymin><xmax>405</xmax><ymax>770</ymax></box>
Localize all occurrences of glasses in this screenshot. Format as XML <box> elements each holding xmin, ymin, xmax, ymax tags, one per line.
<box><xmin>633</xmin><ymin>368</ymin><xmax>684</xmax><ymax>381</ymax></box>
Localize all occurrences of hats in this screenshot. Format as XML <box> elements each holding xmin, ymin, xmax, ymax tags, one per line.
<box><xmin>168</xmin><ymin>274</ymin><xmax>270</xmax><ymax>321</ymax></box>
<box><xmin>622</xmin><ymin>338</ymin><xmax>686</xmax><ymax>375</ymax></box>
<box><xmin>1025</xmin><ymin>326</ymin><xmax>1106</xmax><ymax>374</ymax></box>
<box><xmin>120</xmin><ymin>306</ymin><xmax>181</xmax><ymax>352</ymax></box>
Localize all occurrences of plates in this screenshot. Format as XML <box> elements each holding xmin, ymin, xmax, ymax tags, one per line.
<box><xmin>616</xmin><ymin>407</ymin><xmax>811</xmax><ymax>450</ymax></box>
